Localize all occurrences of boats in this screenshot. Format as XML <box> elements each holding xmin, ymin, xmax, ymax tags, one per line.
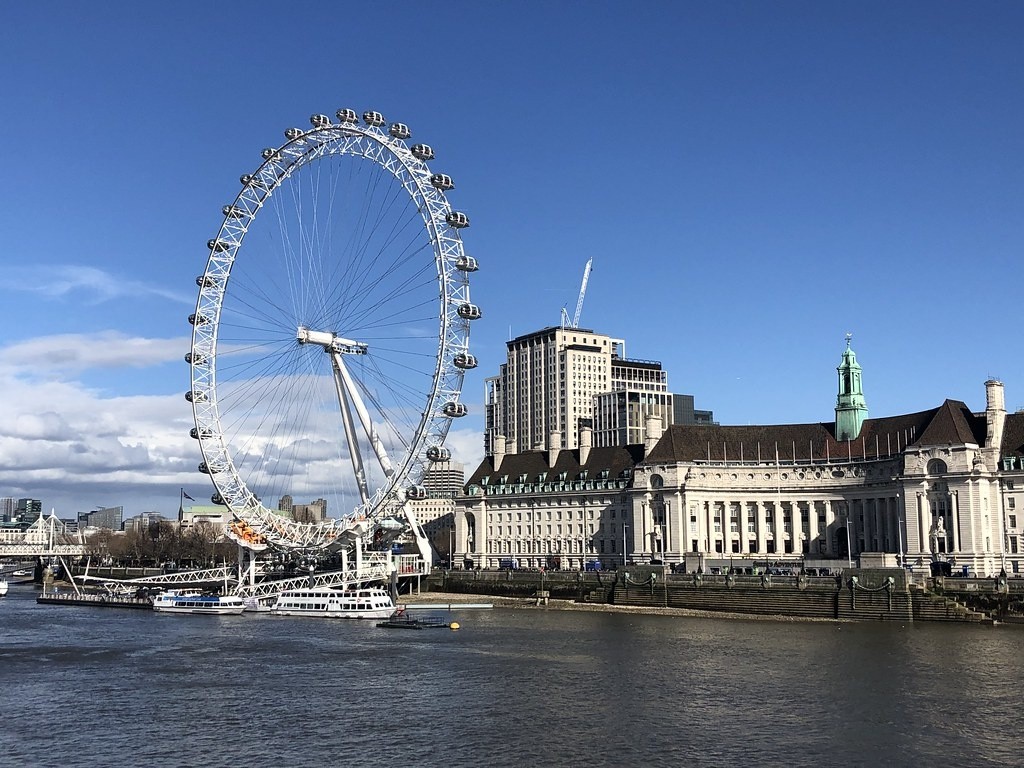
<box><xmin>376</xmin><ymin>607</ymin><xmax>450</xmax><ymax>629</ymax></box>
<box><xmin>0</xmin><ymin>579</ymin><xmax>9</xmax><ymax>594</ymax></box>
<box><xmin>13</xmin><ymin>569</ymin><xmax>25</xmax><ymax>576</ymax></box>
<box><xmin>153</xmin><ymin>594</ymin><xmax>247</xmax><ymax>614</ymax></box>
<box><xmin>270</xmin><ymin>586</ymin><xmax>398</xmax><ymax>619</ymax></box>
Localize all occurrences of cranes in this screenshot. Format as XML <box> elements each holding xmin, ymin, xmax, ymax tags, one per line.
<box><xmin>560</xmin><ymin>255</ymin><xmax>593</xmax><ymax>328</ymax></box>
